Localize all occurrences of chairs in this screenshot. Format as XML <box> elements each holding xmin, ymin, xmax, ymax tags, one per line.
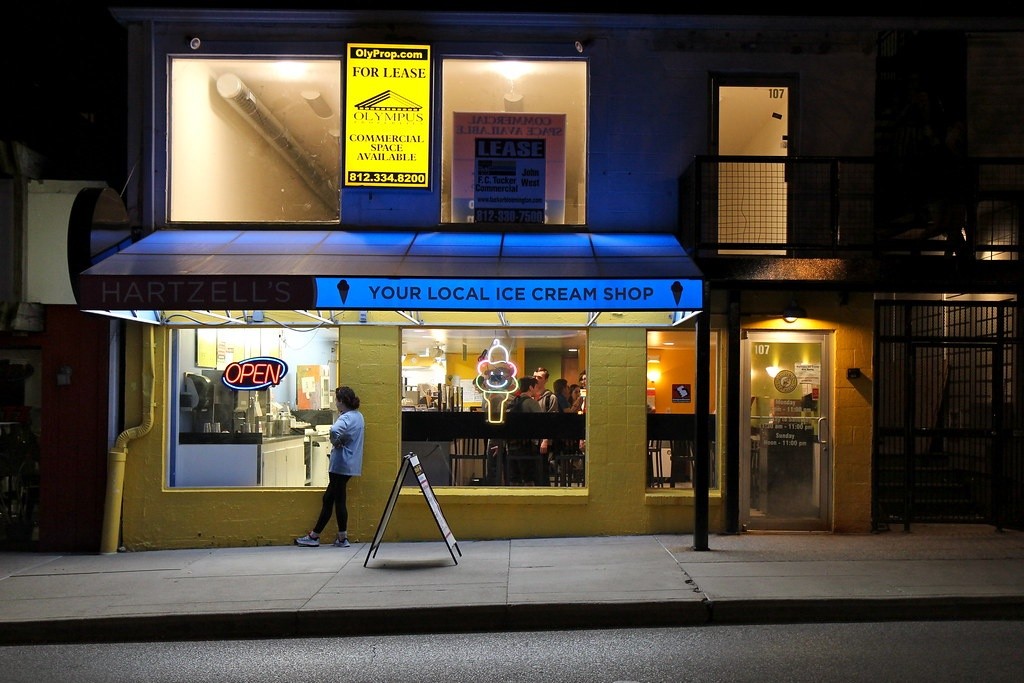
<box><xmin>447</xmin><ymin>438</ymin><xmax>488</xmax><ymax>488</ymax></box>
<box><xmin>645</xmin><ymin>437</ymin><xmax>665</xmax><ymax>490</ymax></box>
<box><xmin>502</xmin><ymin>437</ymin><xmax>546</xmax><ymax>487</ymax></box>
<box><xmin>551</xmin><ymin>436</ymin><xmax>585</xmax><ymax>489</ymax></box>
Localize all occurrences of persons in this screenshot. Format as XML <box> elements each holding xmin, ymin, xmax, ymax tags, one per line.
<box><xmin>567</xmin><ymin>384</ymin><xmax>586</xmax><ymax>455</ymax></box>
<box><xmin>530</xmin><ymin>367</ymin><xmax>558</xmax><ymax>486</ymax></box>
<box><xmin>507</xmin><ymin>375</ymin><xmax>549</xmax><ymax>487</ymax></box>
<box><xmin>553</xmin><ymin>379</ymin><xmax>583</xmax><ymax>470</ymax></box>
<box><xmin>473</xmin><ymin>370</ymin><xmax>516</xmax><ymax>477</ymax></box>
<box><xmin>296</xmin><ymin>386</ymin><xmax>365</xmax><ymax>547</ymax></box>
<box><xmin>578</xmin><ymin>370</ymin><xmax>586</xmax><ymax>413</ymax></box>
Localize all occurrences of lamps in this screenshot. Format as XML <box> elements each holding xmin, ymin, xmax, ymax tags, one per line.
<box><xmin>765</xmin><ymin>366</ymin><xmax>784</xmax><ymax>380</ymax></box>
<box><xmin>645</xmin><ymin>367</ymin><xmax>664</xmax><ymax>387</ymax></box>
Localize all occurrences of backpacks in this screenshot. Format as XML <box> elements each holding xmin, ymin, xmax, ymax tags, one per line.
<box><xmin>504</xmin><ymin>396</ymin><xmax>531</xmax><ymax>450</ymax></box>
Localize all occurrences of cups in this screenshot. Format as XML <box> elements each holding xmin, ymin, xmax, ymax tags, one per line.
<box><xmin>203</xmin><ymin>421</ymin><xmax>252</xmax><ymax>434</ymax></box>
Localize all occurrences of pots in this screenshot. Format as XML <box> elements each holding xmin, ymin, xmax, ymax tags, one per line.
<box><xmin>253</xmin><ymin>414</ymin><xmax>292</xmax><ymax>437</ymax></box>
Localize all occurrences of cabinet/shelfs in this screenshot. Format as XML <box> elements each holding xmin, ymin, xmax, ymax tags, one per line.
<box><xmin>175</xmin><ymin>436</ymin><xmax>306</xmax><ymax>488</ymax></box>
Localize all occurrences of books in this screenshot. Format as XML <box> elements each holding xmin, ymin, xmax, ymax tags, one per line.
<box><xmin>441</xmin><ymin>384</ymin><xmax>463</xmax><ymax>408</ymax></box>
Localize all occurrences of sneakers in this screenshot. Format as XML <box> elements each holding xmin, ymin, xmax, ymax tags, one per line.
<box><xmin>334</xmin><ymin>538</ymin><xmax>351</xmax><ymax>547</ymax></box>
<box><xmin>296</xmin><ymin>534</ymin><xmax>320</xmax><ymax>547</ymax></box>
